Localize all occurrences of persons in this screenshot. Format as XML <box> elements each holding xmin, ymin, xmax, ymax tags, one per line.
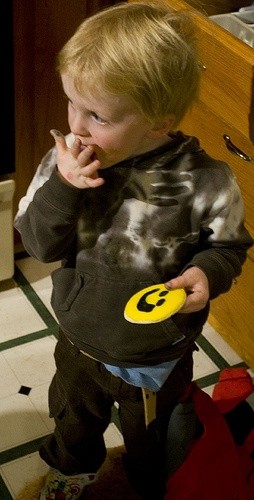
<box><xmin>10</xmin><ymin>3</ymin><xmax>253</xmax><ymax>500</ymax></box>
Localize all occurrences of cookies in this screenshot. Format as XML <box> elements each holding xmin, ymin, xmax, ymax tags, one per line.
<box><xmin>123</xmin><ymin>283</ymin><xmax>187</xmax><ymax>325</ymax></box>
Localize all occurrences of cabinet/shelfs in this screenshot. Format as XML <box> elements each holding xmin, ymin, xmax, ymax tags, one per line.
<box><xmin>122</xmin><ymin>1</ymin><xmax>254</xmax><ymax>377</ymax></box>
